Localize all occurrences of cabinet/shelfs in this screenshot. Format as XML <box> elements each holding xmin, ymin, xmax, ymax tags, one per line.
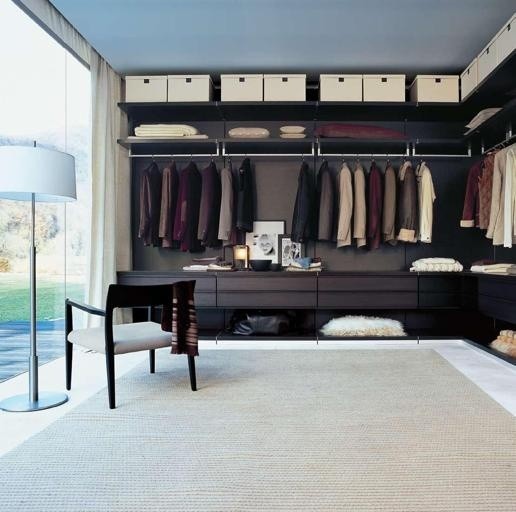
<box><xmin>117</xmin><ymin>101</ymin><xmax>224</xmax><ymax>159</ymax></box>
<box><xmin>317</xmin><ymin>102</ymin><xmax>472</xmax><ymax>157</ymax></box>
<box><xmin>418</xmin><ymin>271</ymin><xmax>475</xmax><ymax>339</ymax></box>
<box><xmin>468</xmin><ymin>272</ymin><xmax>516</xmax><ymax>368</ymax></box>
<box><xmin>317</xmin><ymin>271</ymin><xmax>420</xmax><ymax>343</ymax></box>
<box><xmin>217</xmin><ymin>269</ymin><xmax>318</xmax><ymax>340</ymax></box>
<box><xmin>224</xmin><ymin>101</ymin><xmax>318</xmax><ymax>156</ymax></box>
<box><xmin>118</xmin><ymin>270</ymin><xmax>218</xmax><ymax>340</ymax></box>
<box><xmin>464</xmin><ymin>50</ymin><xmax>516</xmax><ymax>157</ymax></box>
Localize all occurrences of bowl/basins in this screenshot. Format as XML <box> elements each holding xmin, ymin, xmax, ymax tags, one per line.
<box><xmin>249</xmin><ymin>260</ymin><xmax>272</xmax><ymax>271</ymax></box>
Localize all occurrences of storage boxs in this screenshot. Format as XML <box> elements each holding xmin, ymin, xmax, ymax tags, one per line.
<box><xmin>409</xmin><ymin>74</ymin><xmax>460</xmax><ymax>102</ymax></box>
<box><xmin>317</xmin><ymin>74</ymin><xmax>363</xmax><ymax>101</ymax></box>
<box><xmin>461</xmin><ymin>58</ymin><xmax>477</xmax><ymax>99</ymax></box>
<box><xmin>167</xmin><ymin>74</ymin><xmax>215</xmax><ymax>102</ymax></box>
<box><xmin>219</xmin><ymin>75</ymin><xmax>263</xmax><ymax>100</ymax></box>
<box><xmin>124</xmin><ymin>75</ymin><xmax>167</xmax><ymax>102</ymax></box>
<box><xmin>362</xmin><ymin>74</ymin><xmax>405</xmax><ymax>102</ymax></box>
<box><xmin>263</xmin><ymin>73</ymin><xmax>306</xmax><ymax>99</ymax></box>
<box><xmin>477</xmin><ymin>15</ymin><xmax>515</xmax><ymax>86</ymax></box>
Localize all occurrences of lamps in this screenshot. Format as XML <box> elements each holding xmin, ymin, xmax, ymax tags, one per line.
<box><xmin>0</xmin><ymin>139</ymin><xmax>77</xmax><ymax>413</ymax></box>
<box><xmin>232</xmin><ymin>244</ymin><xmax>249</xmax><ymax>271</ymax></box>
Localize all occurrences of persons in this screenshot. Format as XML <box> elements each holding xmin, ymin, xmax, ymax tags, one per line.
<box><xmin>259</xmin><ymin>234</ymin><xmax>275</xmax><ymax>257</ymax></box>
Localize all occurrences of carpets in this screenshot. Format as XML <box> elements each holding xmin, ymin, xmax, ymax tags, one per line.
<box><xmin>1</xmin><ymin>348</ymin><xmax>516</xmax><ymax>512</ymax></box>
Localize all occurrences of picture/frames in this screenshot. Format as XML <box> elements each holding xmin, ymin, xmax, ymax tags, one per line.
<box><xmin>245</xmin><ymin>219</ymin><xmax>286</xmax><ymax>266</ymax></box>
<box><xmin>277</xmin><ymin>234</ymin><xmax>307</xmax><ymax>270</ymax></box>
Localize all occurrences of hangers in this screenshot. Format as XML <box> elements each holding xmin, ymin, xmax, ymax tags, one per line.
<box><xmin>146</xmin><ymin>150</ymin><xmax>253</xmax><ymax>171</ymax></box>
<box><xmin>477</xmin><ymin>132</ymin><xmax>516</xmax><ymax>169</ymax></box>
<box><xmin>298</xmin><ymin>150</ymin><xmax>428</xmax><ymax>177</ymax></box>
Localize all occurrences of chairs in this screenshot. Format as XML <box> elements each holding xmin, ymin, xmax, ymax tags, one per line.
<box><xmin>62</xmin><ymin>279</ymin><xmax>200</xmax><ymax>409</ymax></box>
